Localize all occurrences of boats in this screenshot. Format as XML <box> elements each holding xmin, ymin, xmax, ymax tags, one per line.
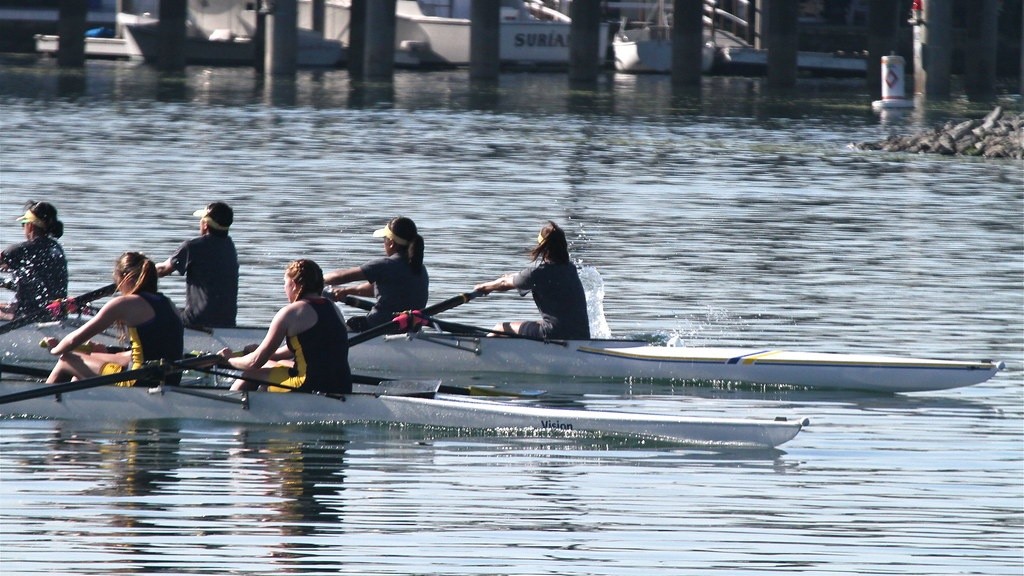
<box><xmin>23</xmin><ymin>0</ymin><xmax>879</xmax><ymax>80</ymax></box>
<box><xmin>1</xmin><ymin>378</ymin><xmax>812</xmax><ymax>457</ymax></box>
<box><xmin>1</xmin><ymin>296</ymin><xmax>1006</xmax><ymax>398</ymax></box>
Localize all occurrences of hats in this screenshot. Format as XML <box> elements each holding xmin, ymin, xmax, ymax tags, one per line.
<box><xmin>373</xmin><ymin>223</ymin><xmax>410</xmax><ymax>246</ymax></box>
<box><xmin>16</xmin><ymin>210</ymin><xmax>47</xmax><ymax>231</ymax></box>
<box><xmin>193</xmin><ymin>207</ymin><xmax>229</xmax><ymax>230</ymax></box>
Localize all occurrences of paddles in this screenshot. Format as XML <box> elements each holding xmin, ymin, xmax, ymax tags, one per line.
<box><xmin>322</xmin><ymin>286</ymin><xmax>487</xmax><ymax>336</ymax></box>
<box><xmin>0</xmin><ymin>362</ymin><xmax>72</xmax><ymax>381</ymax></box>
<box><xmin>348</xmin><ymin>288</ymin><xmax>485</xmax><ymax>349</ymax></box>
<box><xmin>0</xmin><ymin>276</ymin><xmax>101</xmax><ymax>317</ymax></box>
<box><xmin>0</xmin><ymin>350</ymin><xmax>252</xmax><ymax>405</ymax></box>
<box><xmin>0</xmin><ymin>282</ymin><xmax>120</xmax><ymax>335</ymax></box>
<box><xmin>183</xmin><ymin>352</ymin><xmax>546</xmax><ymax>396</ymax></box>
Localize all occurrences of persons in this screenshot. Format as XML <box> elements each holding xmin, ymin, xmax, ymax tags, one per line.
<box><xmin>44</xmin><ymin>251</ymin><xmax>184</xmax><ymax>387</ymax></box>
<box><xmin>0</xmin><ymin>201</ymin><xmax>68</xmax><ymax>322</ymax></box>
<box><xmin>216</xmin><ymin>260</ymin><xmax>352</xmax><ymax>395</ymax></box>
<box><xmin>322</xmin><ymin>217</ymin><xmax>429</xmax><ymax>333</ymax></box>
<box><xmin>154</xmin><ymin>203</ymin><xmax>239</xmax><ymax>326</ymax></box>
<box><xmin>475</xmin><ymin>223</ymin><xmax>591</xmax><ymax>343</ymax></box>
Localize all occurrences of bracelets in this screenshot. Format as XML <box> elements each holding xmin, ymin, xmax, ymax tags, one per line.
<box><xmin>48</xmin><ymin>346</ymin><xmax>55</xmax><ymax>353</ymax></box>
